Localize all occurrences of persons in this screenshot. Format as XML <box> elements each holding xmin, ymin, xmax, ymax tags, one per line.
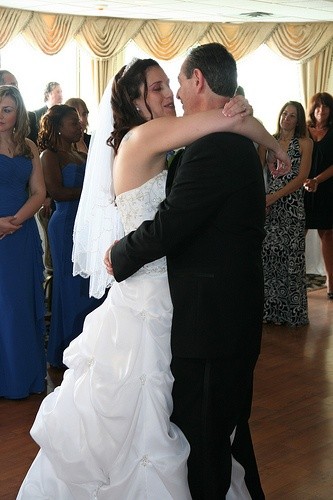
<box><xmin>65</xmin><ymin>97</ymin><xmax>91</xmax><ymax>161</ymax></box>
<box><xmin>16</xmin><ymin>56</ymin><xmax>293</xmax><ymax>500</ymax></box>
<box><xmin>261</xmin><ymin>101</ymin><xmax>313</xmax><ymax>325</ymax></box>
<box><xmin>103</xmin><ymin>41</ymin><xmax>265</xmax><ymax>500</ymax></box>
<box><xmin>303</xmin><ymin>91</ymin><xmax>333</xmax><ymax>300</ymax></box>
<box><xmin>0</xmin><ymin>83</ymin><xmax>47</xmax><ymax>400</ymax></box>
<box><xmin>0</xmin><ymin>70</ymin><xmax>38</xmax><ymax>146</ymax></box>
<box><xmin>38</xmin><ymin>105</ymin><xmax>111</xmax><ymax>373</ymax></box>
<box><xmin>35</xmin><ymin>82</ymin><xmax>62</xmax><ymax>123</ymax></box>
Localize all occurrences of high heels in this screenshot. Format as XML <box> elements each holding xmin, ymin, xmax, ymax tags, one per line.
<box><xmin>326</xmin><ymin>280</ymin><xmax>333</xmax><ymax>299</ymax></box>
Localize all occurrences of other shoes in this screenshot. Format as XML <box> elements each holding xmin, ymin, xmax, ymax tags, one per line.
<box><xmin>263</xmin><ymin>319</ymin><xmax>275</xmax><ymax>328</ymax></box>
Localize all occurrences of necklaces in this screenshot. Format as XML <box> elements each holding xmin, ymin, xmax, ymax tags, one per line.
<box><xmin>7</xmin><ymin>138</ymin><xmax>14</xmax><ymax>155</ymax></box>
